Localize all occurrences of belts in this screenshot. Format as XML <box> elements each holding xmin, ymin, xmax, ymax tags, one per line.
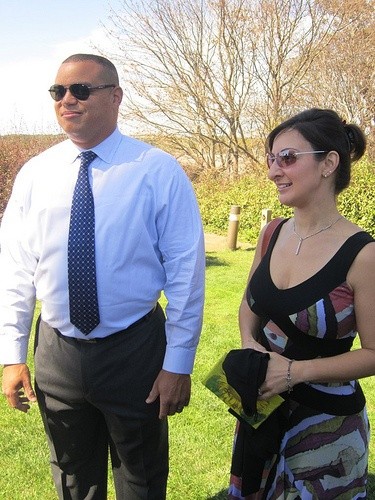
<box><xmin>56</xmin><ymin>303</ymin><xmax>157</xmax><ymax>346</ymax></box>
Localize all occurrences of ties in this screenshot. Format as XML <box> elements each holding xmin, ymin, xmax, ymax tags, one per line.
<box><xmin>67</xmin><ymin>149</ymin><xmax>101</xmax><ymax>336</ymax></box>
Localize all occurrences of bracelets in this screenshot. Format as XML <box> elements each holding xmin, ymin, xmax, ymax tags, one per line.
<box><xmin>287</xmin><ymin>359</ymin><xmax>293</xmax><ymax>392</ymax></box>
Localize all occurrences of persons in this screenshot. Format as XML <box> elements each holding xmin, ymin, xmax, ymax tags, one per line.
<box><xmin>0</xmin><ymin>54</ymin><xmax>205</xmax><ymax>500</ymax></box>
<box><xmin>227</xmin><ymin>108</ymin><xmax>374</xmax><ymax>500</ymax></box>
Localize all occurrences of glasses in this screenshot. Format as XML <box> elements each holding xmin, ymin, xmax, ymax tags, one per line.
<box><xmin>48</xmin><ymin>82</ymin><xmax>116</xmax><ymax>102</ymax></box>
<box><xmin>266</xmin><ymin>148</ymin><xmax>328</xmax><ymax>168</ymax></box>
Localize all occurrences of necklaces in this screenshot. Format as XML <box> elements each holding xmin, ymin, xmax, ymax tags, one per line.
<box><xmin>293</xmin><ymin>217</ymin><xmax>345</xmax><ymax>255</ymax></box>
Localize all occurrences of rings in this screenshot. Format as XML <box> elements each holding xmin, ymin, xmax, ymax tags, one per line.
<box><xmin>257</xmin><ymin>388</ymin><xmax>262</xmax><ymax>396</ymax></box>
